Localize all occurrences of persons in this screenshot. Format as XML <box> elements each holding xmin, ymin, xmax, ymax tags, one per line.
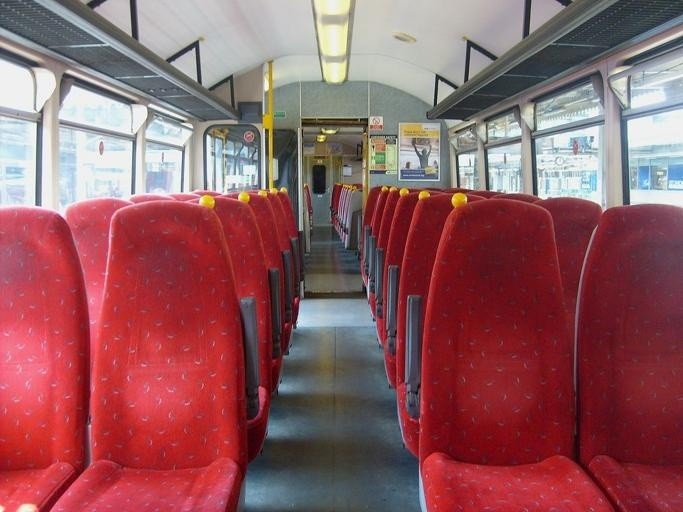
<box><xmin>405</xmin><ymin>141</ymin><xmax>437</xmax><ymax>169</ymax></box>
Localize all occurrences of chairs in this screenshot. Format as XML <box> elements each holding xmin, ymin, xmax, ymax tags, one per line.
<box><xmin>332</xmin><ymin>181</ymin><xmax>681</xmax><ymax>508</ymax></box>
<box><xmin>2</xmin><ymin>188</ymin><xmax>305</xmax><ymax>508</ymax></box>
